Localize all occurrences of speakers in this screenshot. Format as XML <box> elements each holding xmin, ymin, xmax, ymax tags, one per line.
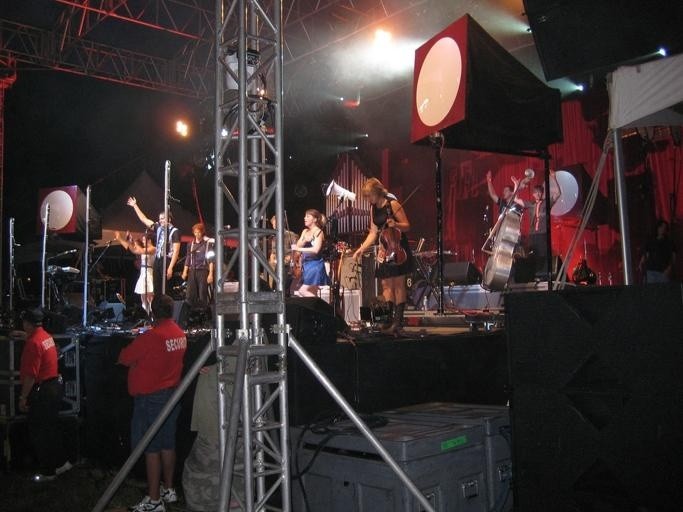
<box><xmin>503</xmin><ymin>285</ymin><xmax>681</xmax><ymax>511</ymax></box>
<box><xmin>542</xmin><ymin>162</ymin><xmax>610</xmax><ymax>226</ymax></box>
<box><xmin>606</xmin><ymin>173</ymin><xmax>651</xmax><ymax>233</ymax></box>
<box><xmin>443</xmin><ymin>261</ymin><xmax>481</xmax><ymax>285</ymax></box>
<box><xmin>521</xmin><ymin>0</ymin><xmax>683</xmax><ymax>82</ymax></box>
<box><xmin>411</xmin><ymin>14</ymin><xmax>564</xmax><ymax>148</ymax></box>
<box><xmin>260</xmin><ymin>295</ymin><xmax>339</xmax><ymax>428</ymax></box>
<box><xmin>35</xmin><ymin>185</ymin><xmax>102</xmax><ymax>241</ymax></box>
<box><xmin>514</xmin><ymin>255</ymin><xmax>570</xmax><ymax>282</ymax></box>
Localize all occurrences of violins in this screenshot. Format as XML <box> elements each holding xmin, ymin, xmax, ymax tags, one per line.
<box><xmin>378</xmin><ymin>202</ymin><xmax>407</xmax><ymax>265</ymax></box>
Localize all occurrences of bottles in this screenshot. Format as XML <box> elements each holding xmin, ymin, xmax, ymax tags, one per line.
<box><xmin>422</xmin><ymin>296</ymin><xmax>429</xmax><ymax>311</ymax></box>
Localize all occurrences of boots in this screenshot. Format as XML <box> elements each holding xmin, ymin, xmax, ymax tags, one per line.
<box><xmin>372</xmin><ymin>301</ymin><xmax>405</xmax><ymax>339</ymax></box>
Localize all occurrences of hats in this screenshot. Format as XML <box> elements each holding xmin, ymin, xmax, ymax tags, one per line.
<box><xmin>20</xmin><ymin>306</ymin><xmax>44</xmax><ymax>322</ymax></box>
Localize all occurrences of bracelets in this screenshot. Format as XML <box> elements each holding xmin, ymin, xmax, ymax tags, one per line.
<box><xmin>18</xmin><ymin>394</ymin><xmax>26</xmax><ymax>400</ymax></box>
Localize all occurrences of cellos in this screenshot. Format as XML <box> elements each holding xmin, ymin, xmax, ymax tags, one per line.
<box><xmin>481</xmin><ymin>168</ymin><xmax>536</xmax><ymax>291</ymax></box>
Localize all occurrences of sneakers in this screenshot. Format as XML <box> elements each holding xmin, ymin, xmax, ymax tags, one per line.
<box><xmin>34</xmin><ymin>461</ymin><xmax>72</xmax><ymax>481</ymax></box>
<box><xmin>155</xmin><ymin>481</ymin><xmax>178</xmax><ymax>504</ymax></box>
<box><xmin>128</xmin><ymin>496</ymin><xmax>165</xmax><ymax>512</ymax></box>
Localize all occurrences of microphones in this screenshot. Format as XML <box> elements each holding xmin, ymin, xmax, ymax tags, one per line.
<box><xmin>65</xmin><ymin>249</ymin><xmax>78</xmax><ymax>254</ymax></box>
<box><xmin>104</xmin><ymin>240</ymin><xmax>113</xmax><ymax>244</ymax></box>
<box><xmin>224</xmin><ymin>224</ymin><xmax>232</xmax><ymax>230</ymax></box>
<box><xmin>149</xmin><ymin>221</ymin><xmax>160</xmax><ymax>228</ymax></box>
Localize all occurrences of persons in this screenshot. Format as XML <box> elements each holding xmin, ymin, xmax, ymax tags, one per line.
<box><xmin>638</xmin><ymin>220</ymin><xmax>677</xmax><ymax>285</ymax></box>
<box><xmin>17</xmin><ymin>307</ymin><xmax>74</xmax><ymax>483</ymax></box>
<box><xmin>351</xmin><ymin>177</ymin><xmax>417</xmax><ymax>334</ymax></box>
<box><xmin>484</xmin><ymin>170</ymin><xmax>524</xmax><ymax>255</ymax></box>
<box><xmin>509</xmin><ymin>168</ymin><xmax>561</xmax><ymax>256</ymax></box>
<box><xmin>268</xmin><ymin>215</ymin><xmax>298</xmax><ymax>296</ymax></box>
<box><xmin>180</xmin><ymin>223</ymin><xmax>215</xmax><ymax>306</ymax></box>
<box><xmin>114</xmin><ymin>230</ymin><xmax>156</xmax><ymax>325</ymax></box>
<box><xmin>117</xmin><ymin>294</ymin><xmax>186</xmax><ymax>511</ymax></box>
<box><xmin>290</xmin><ymin>209</ymin><xmax>333</xmax><ymax>298</ymax></box>
<box><xmin>125</xmin><ymin>196</ymin><xmax>182</xmax><ymax>302</ymax></box>
